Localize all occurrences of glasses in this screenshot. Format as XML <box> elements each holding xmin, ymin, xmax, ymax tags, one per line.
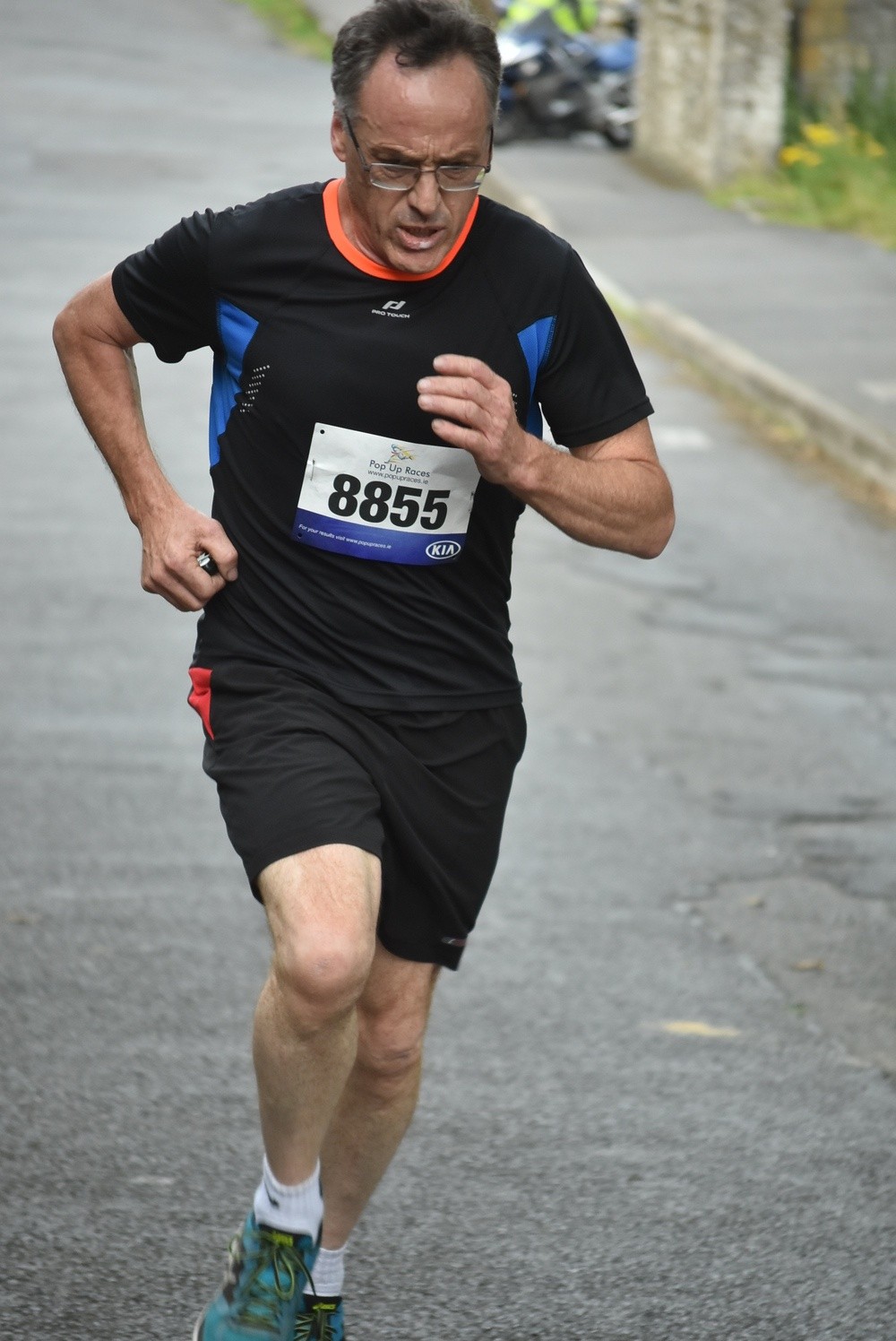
<box><xmin>342</xmin><ymin>109</ymin><xmax>493</xmax><ymax>191</ymax></box>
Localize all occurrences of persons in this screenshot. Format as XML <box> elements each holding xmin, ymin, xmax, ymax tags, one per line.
<box><xmin>50</xmin><ymin>2</ymin><xmax>685</xmax><ymax>1339</ymax></box>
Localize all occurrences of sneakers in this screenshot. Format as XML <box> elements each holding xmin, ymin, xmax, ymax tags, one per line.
<box><xmin>292</xmin><ymin>1292</ymin><xmax>347</xmax><ymax>1341</ymax></box>
<box><xmin>193</xmin><ymin>1186</ymin><xmax>325</xmax><ymax>1341</ymax></box>
